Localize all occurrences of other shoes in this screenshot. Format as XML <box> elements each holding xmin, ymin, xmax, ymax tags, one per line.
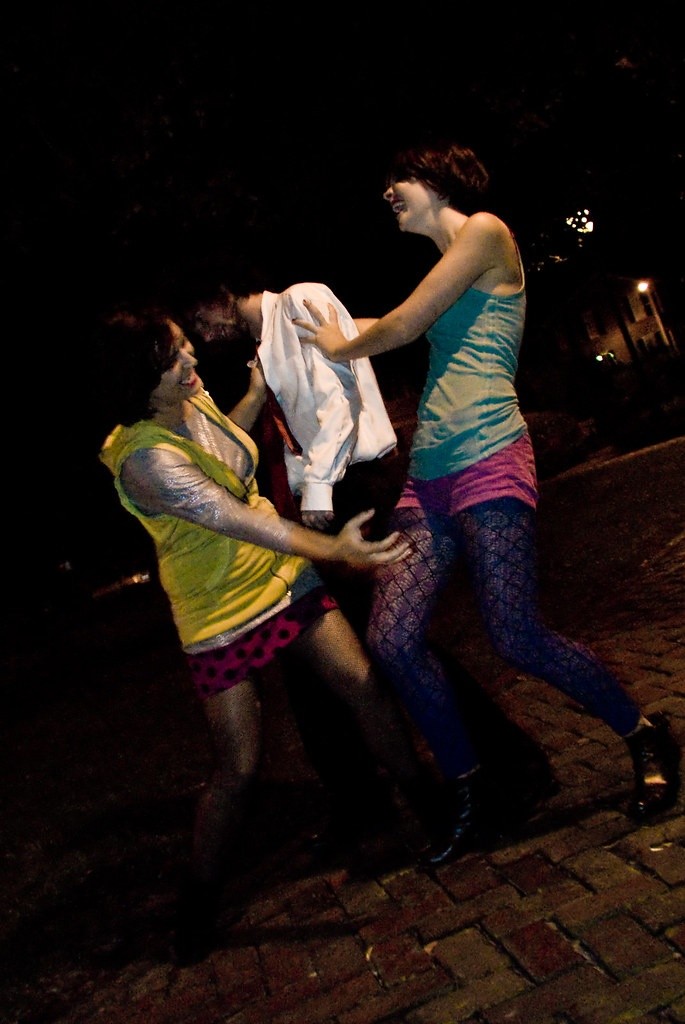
<box><xmin>174</xmin><ymin>873</ymin><xmax>224</xmax><ymax>962</ymax></box>
<box><xmin>362</xmin><ymin>796</ymin><xmax>405</xmax><ymax>876</ymax></box>
<box><xmin>310</xmin><ymin>778</ymin><xmax>381</xmax><ymax>861</ymax></box>
<box><xmin>492</xmin><ymin>735</ymin><xmax>560</xmax><ymax>818</ymax></box>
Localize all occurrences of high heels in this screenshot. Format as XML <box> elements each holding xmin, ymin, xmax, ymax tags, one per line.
<box><xmin>624</xmin><ymin>715</ymin><xmax>681</xmax><ymax>817</ymax></box>
<box><xmin>424</xmin><ymin>766</ymin><xmax>510</xmax><ymax>866</ymax></box>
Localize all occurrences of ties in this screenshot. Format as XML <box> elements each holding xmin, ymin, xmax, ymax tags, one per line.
<box><xmin>255</xmin><ymin>340</ymin><xmax>303</xmax><ymax>456</ymax></box>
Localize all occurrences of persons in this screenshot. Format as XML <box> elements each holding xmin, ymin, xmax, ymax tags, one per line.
<box><xmin>292</xmin><ymin>138</ymin><xmax>680</xmax><ymax>869</ymax></box>
<box><xmin>88</xmin><ymin>252</ymin><xmax>559</xmax><ymax>966</ymax></box>
